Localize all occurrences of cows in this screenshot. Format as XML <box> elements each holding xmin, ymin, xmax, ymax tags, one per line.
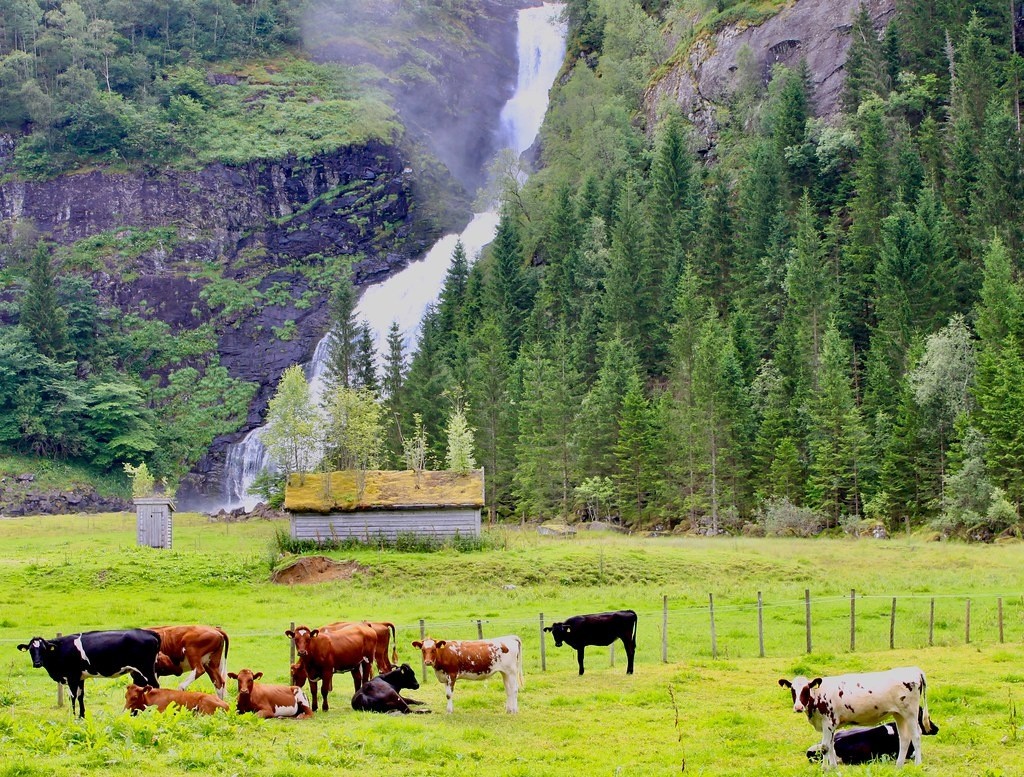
<box><xmin>136</xmin><ymin>625</ymin><xmax>230</xmax><ymax>701</ymax></box>
<box><xmin>412</xmin><ymin>635</ymin><xmax>524</xmax><ymax>714</ymax></box>
<box><xmin>227</xmin><ymin>668</ymin><xmax>313</xmax><ymax>720</ymax></box>
<box><xmin>778</xmin><ymin>666</ymin><xmax>931</xmax><ymax>770</ymax></box>
<box><xmin>16</xmin><ymin>629</ymin><xmax>162</xmax><ymax>718</ymax></box>
<box><xmin>806</xmin><ymin>705</ymin><xmax>939</xmax><ymax>764</ymax></box>
<box><xmin>290</xmin><ymin>621</ymin><xmax>399</xmax><ymax>691</ymax></box>
<box><xmin>285</xmin><ymin>622</ymin><xmax>378</xmax><ymax>712</ymax></box>
<box><xmin>125</xmin><ymin>684</ymin><xmax>229</xmax><ymax>715</ymax></box>
<box><xmin>543</xmin><ymin>610</ymin><xmax>638</xmax><ymax>675</ymax></box>
<box><xmin>352</xmin><ymin>663</ymin><xmax>432</xmax><ymax>715</ymax></box>
